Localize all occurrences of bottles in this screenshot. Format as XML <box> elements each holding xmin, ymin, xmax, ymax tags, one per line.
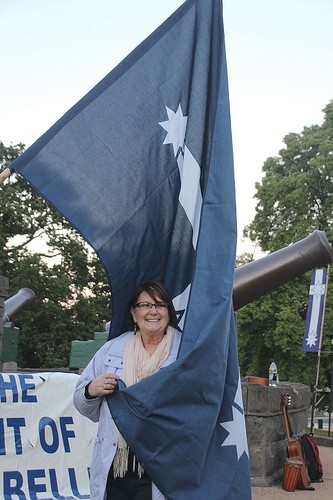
<box><xmin>269</xmin><ymin>362</ymin><xmax>277</xmax><ymax>387</ymax></box>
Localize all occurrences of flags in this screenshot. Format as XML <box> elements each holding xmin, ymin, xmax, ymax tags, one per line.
<box><xmin>10</xmin><ymin>0</ymin><xmax>257</xmax><ymax>499</ymax></box>
<box><xmin>300</xmin><ymin>266</ymin><xmax>328</xmax><ymax>354</ymax></box>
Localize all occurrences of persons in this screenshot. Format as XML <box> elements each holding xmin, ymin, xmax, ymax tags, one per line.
<box><xmin>69</xmin><ymin>279</ymin><xmax>185</xmax><ymax>500</ymax></box>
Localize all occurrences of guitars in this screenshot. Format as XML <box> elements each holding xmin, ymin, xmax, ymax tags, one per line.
<box><xmin>281</xmin><ymin>392</ymin><xmax>311</xmax><ymax>489</ymax></box>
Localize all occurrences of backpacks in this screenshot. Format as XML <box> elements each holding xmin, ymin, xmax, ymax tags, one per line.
<box><xmin>300</xmin><ymin>432</ymin><xmax>324</xmax><ymax>483</ymax></box>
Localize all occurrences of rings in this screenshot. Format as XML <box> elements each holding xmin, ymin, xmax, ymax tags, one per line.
<box><xmin>105</xmin><ymin>378</ymin><xmax>108</xmax><ymax>384</ymax></box>
<box><xmin>104</xmin><ymin>384</ymin><xmax>107</xmax><ymax>390</ymax></box>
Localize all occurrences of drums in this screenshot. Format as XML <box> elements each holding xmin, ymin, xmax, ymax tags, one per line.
<box><xmin>283</xmin><ymin>458</ymin><xmax>303</xmax><ymax>491</ymax></box>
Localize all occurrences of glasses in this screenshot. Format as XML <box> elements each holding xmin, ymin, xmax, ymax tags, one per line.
<box><xmin>133</xmin><ymin>302</ymin><xmax>168</xmax><ymax>310</ymax></box>
